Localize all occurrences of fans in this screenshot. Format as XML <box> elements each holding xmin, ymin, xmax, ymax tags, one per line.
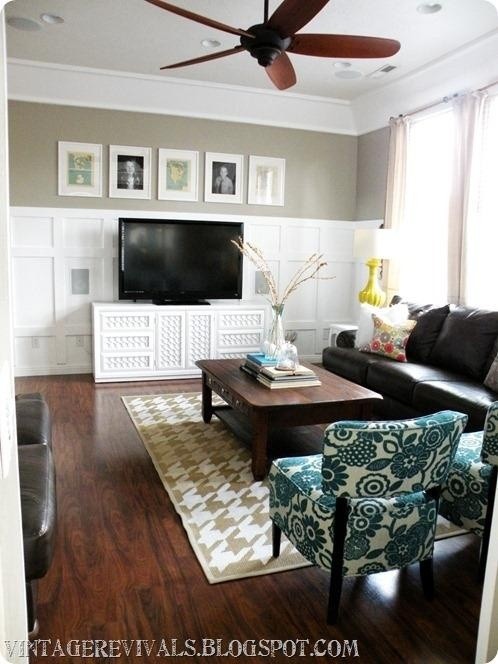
<box><xmin>145</xmin><ymin>0</ymin><xmax>401</xmax><ymax>90</ymax></box>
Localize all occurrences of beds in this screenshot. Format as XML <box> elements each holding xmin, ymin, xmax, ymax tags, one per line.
<box><xmin>194</xmin><ymin>353</ymin><xmax>384</xmax><ymax>482</ymax></box>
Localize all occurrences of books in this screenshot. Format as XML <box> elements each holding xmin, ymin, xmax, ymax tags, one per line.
<box><xmin>241</xmin><ymin>352</ymin><xmax>293</xmax><ymax>378</ymax></box>
<box><xmin>256</xmin><ymin>365</ymin><xmax>321</xmax><ymax>389</ymax></box>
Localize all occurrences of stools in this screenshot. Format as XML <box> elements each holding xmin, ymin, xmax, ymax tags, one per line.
<box><xmin>14</xmin><ymin>391</ymin><xmax>57</xmax><ymax>632</ymax></box>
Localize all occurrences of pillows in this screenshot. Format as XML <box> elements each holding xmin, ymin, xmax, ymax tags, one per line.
<box><xmin>427</xmin><ymin>312</ymin><xmax>498</xmax><ymax>383</ymax></box>
<box><xmin>405</xmin><ymin>304</ymin><xmax>450</xmax><ymax>364</ymax></box>
<box><xmin>358</xmin><ymin>314</ymin><xmax>418</xmax><ymax>362</ymax></box>
<box><xmin>483</xmin><ymin>350</ymin><xmax>498</xmax><ymax>394</ymax></box>
<box><xmin>354</xmin><ymin>303</ymin><xmax>408</xmax><ymax>349</ymax></box>
<box><xmin>389</xmin><ymin>295</ymin><xmax>432</xmax><ymax>320</ymax></box>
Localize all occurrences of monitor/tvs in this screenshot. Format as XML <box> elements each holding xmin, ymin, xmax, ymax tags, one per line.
<box><xmin>118</xmin><ymin>217</ymin><xmax>245</xmax><ymax>306</ymax></box>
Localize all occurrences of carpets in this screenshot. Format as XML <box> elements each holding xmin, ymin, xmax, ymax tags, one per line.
<box><xmin>121</xmin><ymin>391</ymin><xmax>471</xmax><ymax>585</ymax></box>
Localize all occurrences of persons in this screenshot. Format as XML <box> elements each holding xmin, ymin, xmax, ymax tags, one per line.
<box><xmin>120</xmin><ymin>160</ymin><xmax>141</xmax><ymax>190</ymax></box>
<box><xmin>214</xmin><ymin>166</ymin><xmax>234</xmax><ymax>194</ymax></box>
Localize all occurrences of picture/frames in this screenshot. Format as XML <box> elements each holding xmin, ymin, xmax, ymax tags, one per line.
<box><xmin>58</xmin><ymin>141</ymin><xmax>286</xmax><ymax>207</ymax></box>
<box><xmin>264</xmin><ymin>304</ymin><xmax>287</xmax><ymax>361</ymax></box>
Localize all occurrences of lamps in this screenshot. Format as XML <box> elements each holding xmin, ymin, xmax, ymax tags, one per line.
<box><xmin>350</xmin><ymin>229</ymin><xmax>399</xmax><ymax>308</ymax></box>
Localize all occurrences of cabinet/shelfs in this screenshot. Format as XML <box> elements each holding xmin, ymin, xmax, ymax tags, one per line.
<box><xmin>91</xmin><ymin>303</ymin><xmax>270</xmax><ymax>384</ymax></box>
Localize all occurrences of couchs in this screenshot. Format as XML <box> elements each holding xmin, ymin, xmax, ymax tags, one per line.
<box><xmin>323</xmin><ymin>329</ymin><xmax>498</xmax><ymax>432</ymax></box>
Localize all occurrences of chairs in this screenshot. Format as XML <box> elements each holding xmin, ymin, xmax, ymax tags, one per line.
<box><xmin>438</xmin><ymin>400</ymin><xmax>498</xmax><ymax>584</ymax></box>
<box><xmin>268</xmin><ymin>409</ymin><xmax>468</xmax><ymax>627</ymax></box>
